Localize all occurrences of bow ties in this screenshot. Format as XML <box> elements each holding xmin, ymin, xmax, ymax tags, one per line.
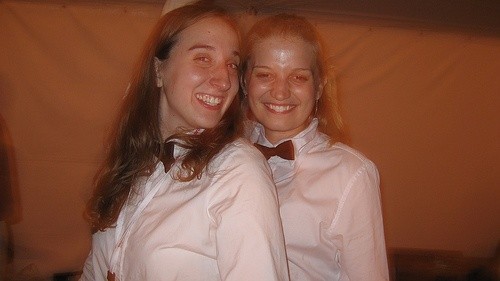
<box><xmin>252</xmin><ymin>140</ymin><xmax>295</xmax><ymax>160</ymax></box>
<box><xmin>150</xmin><ymin>135</ymin><xmax>176</xmax><ymax>173</ymax></box>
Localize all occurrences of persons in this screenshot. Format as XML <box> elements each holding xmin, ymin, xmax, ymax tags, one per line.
<box><xmin>77</xmin><ymin>0</ymin><xmax>390</xmax><ymax>281</ymax></box>
